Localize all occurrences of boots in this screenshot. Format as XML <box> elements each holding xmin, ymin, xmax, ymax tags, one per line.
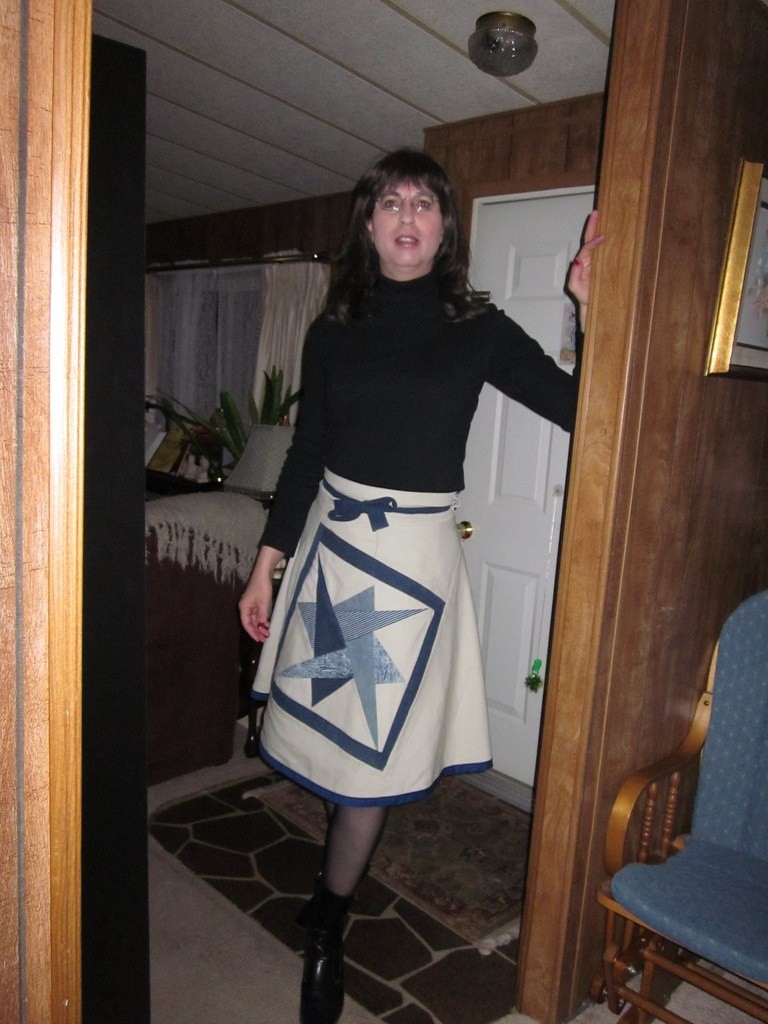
<box><xmin>299</xmin><ymin>883</ymin><xmax>348</xmax><ymax>1024</ymax></box>
<box><xmin>293</xmin><ymin>861</ymin><xmax>325</xmax><ymax>891</ymax></box>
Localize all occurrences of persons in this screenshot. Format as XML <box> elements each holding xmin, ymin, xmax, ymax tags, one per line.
<box><xmin>238</xmin><ymin>149</ymin><xmax>604</xmax><ymax>1024</ymax></box>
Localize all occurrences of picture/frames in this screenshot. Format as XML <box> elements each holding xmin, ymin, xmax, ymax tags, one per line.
<box><xmin>705</xmin><ymin>160</ymin><xmax>768</xmax><ymax>381</ymax></box>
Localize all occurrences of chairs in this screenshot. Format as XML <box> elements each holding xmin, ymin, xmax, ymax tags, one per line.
<box><xmin>596</xmin><ymin>592</ymin><xmax>768</xmax><ymax>1024</ymax></box>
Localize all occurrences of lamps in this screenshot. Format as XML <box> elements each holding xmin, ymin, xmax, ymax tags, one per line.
<box><xmin>223</xmin><ymin>423</ymin><xmax>296</xmax><ymax>507</ymax></box>
<box><xmin>468</xmin><ymin>11</ymin><xmax>538</xmax><ymax>78</ymax></box>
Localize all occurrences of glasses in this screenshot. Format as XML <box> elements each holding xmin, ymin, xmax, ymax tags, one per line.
<box><xmin>371</xmin><ymin>193</ymin><xmax>441</xmax><ymax>214</ymax></box>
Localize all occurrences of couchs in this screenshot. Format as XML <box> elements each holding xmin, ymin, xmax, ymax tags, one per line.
<box><xmin>146</xmin><ymin>491</ymin><xmax>269</xmax><ymax>788</ymax></box>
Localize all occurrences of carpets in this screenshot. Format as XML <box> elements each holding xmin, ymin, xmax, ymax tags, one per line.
<box><xmin>256</xmin><ymin>776</ymin><xmax>532</xmax><ymax>952</ymax></box>
